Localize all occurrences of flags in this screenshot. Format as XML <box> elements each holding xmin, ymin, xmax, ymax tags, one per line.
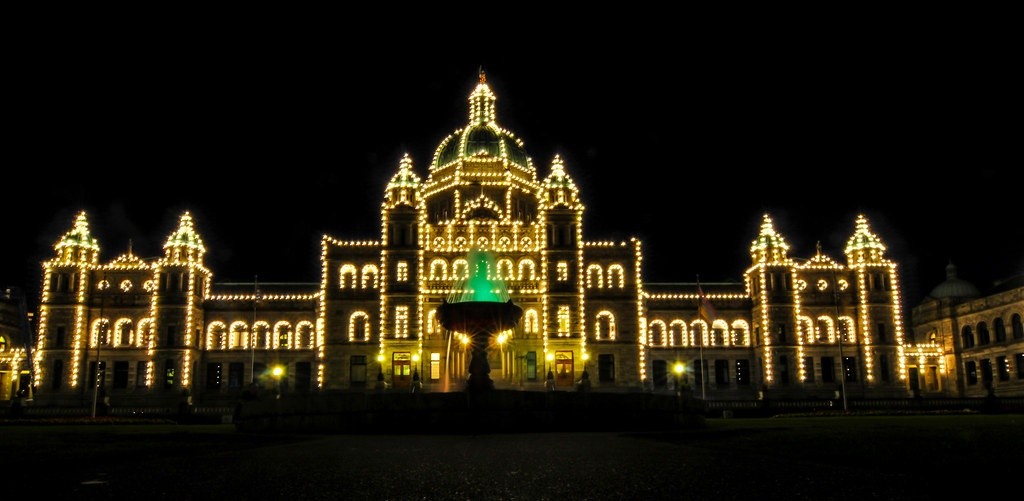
<box><xmin>697</xmin><ymin>286</ymin><xmax>717</xmax><ymax>328</ymax></box>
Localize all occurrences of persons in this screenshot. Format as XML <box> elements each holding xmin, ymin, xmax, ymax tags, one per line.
<box><xmin>375</xmin><ymin>372</ymin><xmax>388</xmax><ymax>392</ymax></box>
<box><xmin>4</xmin><ymin>372</ymin><xmax>37</xmax><ymax>426</ymax></box>
<box><xmin>103</xmin><ymin>388</ymin><xmax>111</xmax><ymax>417</ymax></box>
<box><xmin>547</xmin><ymin>371</ymin><xmax>555</xmax><ymax>392</ymax></box>
<box><xmin>241</xmin><ymin>382</ymin><xmax>260</xmax><ymax>402</ymax></box>
<box><xmin>278</xmin><ymin>375</ymin><xmax>290</xmax><ymax>395</ymax></box>
<box><xmin>171</xmin><ymin>389</ymin><xmax>192</xmax><ymax>424</ymax></box>
<box><xmin>410</xmin><ymin>371</ymin><xmax>423</xmax><ymax>393</ymax></box>
<box><xmin>681</xmin><ymin>376</ymin><xmax>692</xmax><ymax>405</ymax></box>
<box><xmin>580</xmin><ymin>371</ymin><xmax>592</xmax><ymax>391</ymax></box>
